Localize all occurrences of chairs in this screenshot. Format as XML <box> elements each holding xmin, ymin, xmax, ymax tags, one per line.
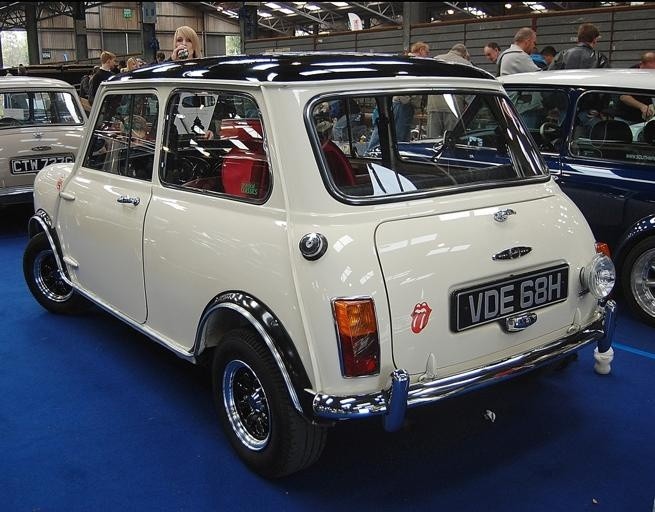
<box><xmin>518</xmin><ymin>110</ymin><xmax>655</xmax><ymax>154</ymax></box>
<box><xmin>146</xmin><ymin>121</ymin><xmax>357</xmax><ymax>198</ymax></box>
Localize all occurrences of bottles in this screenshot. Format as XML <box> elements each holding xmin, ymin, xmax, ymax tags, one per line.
<box><xmin>177</xmin><ymin>46</ymin><xmax>189</xmax><ymax>60</ymax></box>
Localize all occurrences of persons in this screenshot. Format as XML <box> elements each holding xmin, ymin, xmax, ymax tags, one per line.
<box><xmin>81</xmin><ymin>49</ymin><xmax>166</xmax><ymax>158</ymax></box>
<box><xmin>362</xmin><ymin>21</ymin><xmax>655</xmax><ymax>162</ymax></box>
<box><xmin>321</xmin><ymin>96</ymin><xmax>365</xmax><ymax>141</ymax></box>
<box><xmin>164</xmin><ymin>25</ymin><xmax>237</xmax><ymax>143</ymax></box>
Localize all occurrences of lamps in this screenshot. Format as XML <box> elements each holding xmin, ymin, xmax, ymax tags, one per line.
<box><xmin>221</xmin><ymin>1</ymin><xmax>348</xmax><ymax>18</ymax></box>
